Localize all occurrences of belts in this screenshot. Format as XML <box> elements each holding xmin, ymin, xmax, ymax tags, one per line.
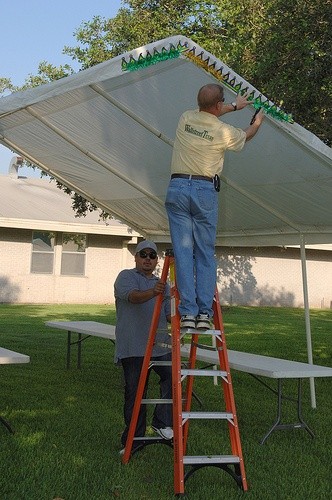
<box><xmin>171</xmin><ymin>173</ymin><xmax>213</xmax><ymax>183</ymax></box>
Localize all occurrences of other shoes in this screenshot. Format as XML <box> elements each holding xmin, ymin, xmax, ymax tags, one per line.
<box><xmin>196</xmin><ymin>313</ymin><xmax>211</xmax><ymax>329</ymax></box>
<box><xmin>180</xmin><ymin>315</ymin><xmax>196</xmax><ymax>329</ymax></box>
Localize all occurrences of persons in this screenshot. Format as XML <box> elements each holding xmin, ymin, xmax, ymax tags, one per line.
<box><xmin>165</xmin><ymin>83</ymin><xmax>268</xmax><ymax>330</ymax></box>
<box><xmin>114</xmin><ymin>241</ymin><xmax>174</xmax><ymax>454</ymax></box>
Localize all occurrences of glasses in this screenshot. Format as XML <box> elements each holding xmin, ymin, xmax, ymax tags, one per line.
<box><xmin>221</xmin><ymin>98</ymin><xmax>225</xmax><ymax>102</ymax></box>
<box><xmin>139</xmin><ymin>251</ymin><xmax>157</xmax><ymax>259</ymax></box>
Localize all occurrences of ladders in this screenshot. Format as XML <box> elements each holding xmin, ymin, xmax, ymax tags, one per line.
<box><xmin>122</xmin><ymin>242</ymin><xmax>248</xmax><ymax>496</ymax></box>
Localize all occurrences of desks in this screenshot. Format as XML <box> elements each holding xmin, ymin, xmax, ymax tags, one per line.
<box><xmin>0</xmin><ymin>346</ymin><xmax>32</xmax><ymax>437</ymax></box>
<box><xmin>47</xmin><ymin>320</ymin><xmax>332</xmax><ymax>445</ymax></box>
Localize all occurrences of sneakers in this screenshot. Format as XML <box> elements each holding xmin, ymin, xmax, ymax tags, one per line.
<box><xmin>152</xmin><ymin>425</ymin><xmax>175</xmax><ymax>441</ymax></box>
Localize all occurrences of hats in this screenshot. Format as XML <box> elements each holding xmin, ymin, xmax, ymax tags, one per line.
<box><xmin>134</xmin><ymin>240</ymin><xmax>158</xmax><ymax>254</ymax></box>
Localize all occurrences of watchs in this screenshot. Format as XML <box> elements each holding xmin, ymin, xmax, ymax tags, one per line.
<box><xmin>232</xmin><ymin>102</ymin><xmax>237</xmax><ymax>111</ymax></box>
